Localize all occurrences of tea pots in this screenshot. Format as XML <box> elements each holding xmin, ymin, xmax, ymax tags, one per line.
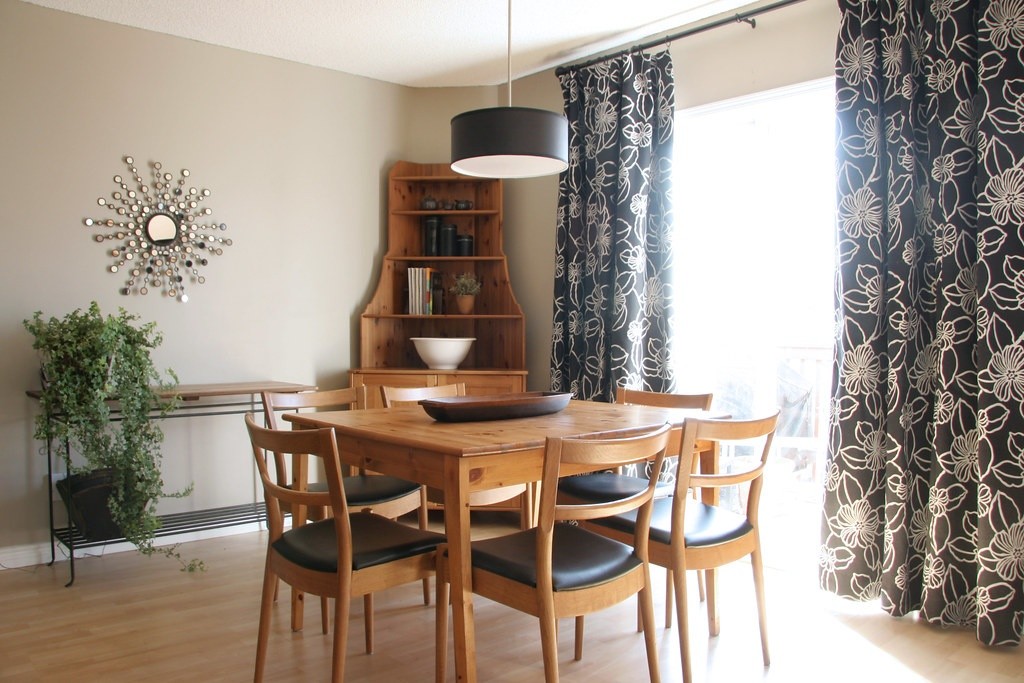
<box><xmin>416</xmin><ymin>195</ymin><xmax>445</xmax><ymax>210</ymax></box>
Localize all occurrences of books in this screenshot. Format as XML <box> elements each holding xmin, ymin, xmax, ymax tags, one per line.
<box><xmin>408</xmin><ymin>267</ymin><xmax>449</xmax><ymax>315</ymax></box>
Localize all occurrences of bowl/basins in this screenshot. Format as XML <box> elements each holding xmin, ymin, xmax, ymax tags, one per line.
<box><xmin>409</xmin><ymin>337</ymin><xmax>477</xmax><ymax>369</ymax></box>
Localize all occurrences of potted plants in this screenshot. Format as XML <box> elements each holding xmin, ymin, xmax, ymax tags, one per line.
<box><xmin>447</xmin><ymin>270</ymin><xmax>481</xmax><ymax>314</ymax></box>
<box><xmin>22</xmin><ymin>299</ymin><xmax>207</xmax><ymax>575</ymax></box>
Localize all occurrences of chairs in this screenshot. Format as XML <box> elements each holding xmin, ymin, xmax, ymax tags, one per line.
<box><xmin>244</xmin><ymin>411</ymin><xmax>451</xmax><ymax>683</ymax></box>
<box><xmin>574</xmin><ymin>407</ymin><xmax>783</xmax><ymax>683</ymax></box>
<box><xmin>435</xmin><ymin>420</ymin><xmax>674</xmax><ymax>682</ymax></box>
<box><xmin>379</xmin><ymin>382</ymin><xmax>534</xmax><ymax>530</ymax></box>
<box><xmin>259</xmin><ymin>382</ymin><xmax>431</xmax><ymax>636</ymax></box>
<box><xmin>556</xmin><ymin>387</ymin><xmax>714</xmax><ymax>634</ymax></box>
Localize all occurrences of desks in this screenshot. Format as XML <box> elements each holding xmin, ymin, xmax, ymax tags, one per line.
<box><xmin>280</xmin><ymin>400</ymin><xmax>733</xmax><ymax>683</ymax></box>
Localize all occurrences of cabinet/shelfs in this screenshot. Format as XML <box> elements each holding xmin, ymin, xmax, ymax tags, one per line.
<box><xmin>359</xmin><ymin>159</ymin><xmax>526</xmax><ymax>371</ymax></box>
<box><xmin>26</xmin><ymin>380</ymin><xmax>319</xmax><ymax>588</ymax></box>
<box><xmin>347</xmin><ymin>367</ymin><xmax>540</xmax><ymax>530</ymax></box>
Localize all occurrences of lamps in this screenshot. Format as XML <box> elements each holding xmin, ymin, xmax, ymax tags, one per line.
<box><xmin>450</xmin><ymin>1</ymin><xmax>568</xmax><ymax>179</ymax></box>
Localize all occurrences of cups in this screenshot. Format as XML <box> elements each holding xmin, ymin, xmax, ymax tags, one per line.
<box><xmin>440</xmin><ymin>224</ymin><xmax>458</xmax><ymax>256</ymax></box>
<box><xmin>422</xmin><ymin>215</ymin><xmax>442</xmax><ymax>256</ymax></box>
<box><xmin>455</xmin><ymin>199</ymin><xmax>474</xmax><ymax>210</ymax></box>
<box><xmin>457</xmin><ymin>235</ymin><xmax>474</xmax><ymax>257</ymax></box>
